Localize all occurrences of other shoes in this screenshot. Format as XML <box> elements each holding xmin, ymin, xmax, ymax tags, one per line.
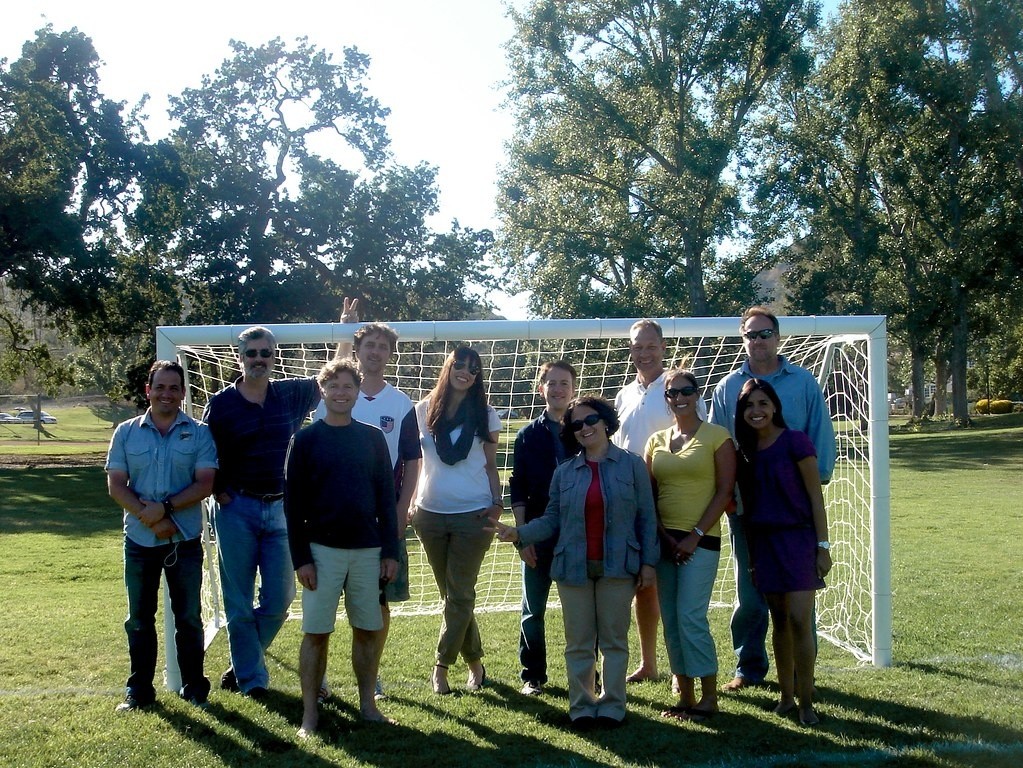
<box><xmin>721</xmin><ymin>676</ymin><xmax>750</xmax><ymax>691</ymax></box>
<box><xmin>801</xmin><ymin>714</ymin><xmax>819</xmax><ymax>726</ymax></box>
<box><xmin>521</xmin><ymin>680</ymin><xmax>542</xmax><ymax>696</ymax></box>
<box><xmin>190</xmin><ymin>696</ymin><xmax>211</xmax><ymax>709</ymax></box>
<box><xmin>221</xmin><ymin>663</ymin><xmax>237</xmax><ymax>690</ymax></box>
<box><xmin>373</xmin><ymin>675</ymin><xmax>389</xmax><ymax>700</ymax></box>
<box><xmin>795</xmin><ymin>682</ymin><xmax>820</xmax><ymax>700</ymax></box>
<box><xmin>115</xmin><ymin>690</ymin><xmax>156</xmax><ymax>711</ymax></box>
<box><xmin>595</xmin><ymin>681</ymin><xmax>602</xmax><ymax>695</ymax></box>
<box><xmin>779</xmin><ymin>701</ymin><xmax>797</xmax><ymax>718</ymax></box>
<box><xmin>317</xmin><ymin>674</ymin><xmax>331</xmax><ymax>703</ymax></box>
<box><xmin>248</xmin><ymin>687</ymin><xmax>267</xmax><ymax>699</ymax></box>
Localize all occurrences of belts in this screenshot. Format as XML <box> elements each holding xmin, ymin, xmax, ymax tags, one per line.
<box><xmin>242</xmin><ymin>490</ymin><xmax>284</xmax><ymax>503</ymax></box>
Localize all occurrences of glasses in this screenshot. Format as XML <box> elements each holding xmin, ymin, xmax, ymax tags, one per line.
<box><xmin>664</xmin><ymin>386</ymin><xmax>697</xmax><ymax>398</ymax></box>
<box><xmin>379</xmin><ymin>576</ymin><xmax>389</xmax><ymax>607</ymax></box>
<box><xmin>743</xmin><ymin>329</ymin><xmax>776</xmax><ymax>340</ymax></box>
<box><xmin>245</xmin><ymin>348</ymin><xmax>273</xmax><ymax>358</ymax></box>
<box><xmin>572</xmin><ymin>414</ymin><xmax>602</xmax><ymax>432</ymax></box>
<box><xmin>453</xmin><ymin>360</ymin><xmax>480</xmax><ymax>375</ymax></box>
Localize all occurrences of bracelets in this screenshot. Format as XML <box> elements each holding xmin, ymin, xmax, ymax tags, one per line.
<box><xmin>161</xmin><ymin>499</ymin><xmax>175</xmax><ymax>517</ymax></box>
<box><xmin>694</xmin><ymin>527</ymin><xmax>705</xmax><ymax>537</ymax></box>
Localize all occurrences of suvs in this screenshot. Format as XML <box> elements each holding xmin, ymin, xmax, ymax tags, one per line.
<box><xmin>14</xmin><ymin>407</ymin><xmax>57</xmax><ymax>424</ymax></box>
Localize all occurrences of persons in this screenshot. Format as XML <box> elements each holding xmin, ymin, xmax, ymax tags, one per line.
<box><xmin>405</xmin><ymin>346</ymin><xmax>503</xmax><ymax>698</ymax></box>
<box><xmin>199</xmin><ymin>297</ymin><xmax>360</xmax><ymax>702</ymax></box>
<box><xmin>104</xmin><ymin>360</ymin><xmax>219</xmax><ymax>713</ymax></box>
<box><xmin>283</xmin><ymin>358</ymin><xmax>402</xmax><ymax>744</ymax></box>
<box><xmin>708</xmin><ymin>306</ymin><xmax>838</xmax><ymax>695</ymax></box>
<box><xmin>308</xmin><ymin>325</ymin><xmax>422</xmax><ymax>709</ymax></box>
<box><xmin>726</xmin><ymin>379</ymin><xmax>832</xmax><ymax>725</ymax></box>
<box><xmin>508</xmin><ymin>361</ymin><xmax>600</xmax><ymax>696</ymax></box>
<box><xmin>609</xmin><ymin>318</ymin><xmax>708</xmax><ymax>697</ymax></box>
<box><xmin>646</xmin><ymin>372</ymin><xmax>738</xmax><ymax>718</ymax></box>
<box><xmin>484</xmin><ymin>396</ymin><xmax>660</xmax><ymax>730</ymax></box>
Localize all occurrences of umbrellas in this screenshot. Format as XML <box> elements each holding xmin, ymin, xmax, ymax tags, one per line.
<box><xmin>13</xmin><ymin>406</ymin><xmax>26</xmax><ymax>411</ymax></box>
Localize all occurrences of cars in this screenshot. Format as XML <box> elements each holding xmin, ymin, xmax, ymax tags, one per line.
<box><xmin>0</xmin><ymin>413</ymin><xmax>21</xmax><ymax>421</ymax></box>
<box><xmin>496</xmin><ymin>410</ymin><xmax>518</xmax><ymax>419</ymax></box>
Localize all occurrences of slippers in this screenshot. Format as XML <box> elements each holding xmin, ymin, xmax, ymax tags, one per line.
<box><xmin>626</xmin><ymin>673</ymin><xmax>643</xmax><ymax>681</ymax></box>
<box><xmin>661</xmin><ymin>701</ymin><xmax>720</xmax><ymax>724</ymax></box>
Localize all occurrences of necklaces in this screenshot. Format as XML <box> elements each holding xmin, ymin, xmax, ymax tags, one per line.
<box><xmin>586</xmin><ymin>454</ymin><xmax>606</xmax><ymax>461</ymax></box>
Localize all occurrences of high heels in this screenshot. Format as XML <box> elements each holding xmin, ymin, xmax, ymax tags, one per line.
<box><xmin>465</xmin><ymin>664</ymin><xmax>485</xmax><ymax>689</ymax></box>
<box><xmin>431</xmin><ymin>663</ymin><xmax>451</xmax><ymax>694</ymax></box>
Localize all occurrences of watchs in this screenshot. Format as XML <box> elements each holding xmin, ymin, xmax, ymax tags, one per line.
<box><xmin>818</xmin><ymin>541</ymin><xmax>830</xmax><ymax>549</ymax></box>
